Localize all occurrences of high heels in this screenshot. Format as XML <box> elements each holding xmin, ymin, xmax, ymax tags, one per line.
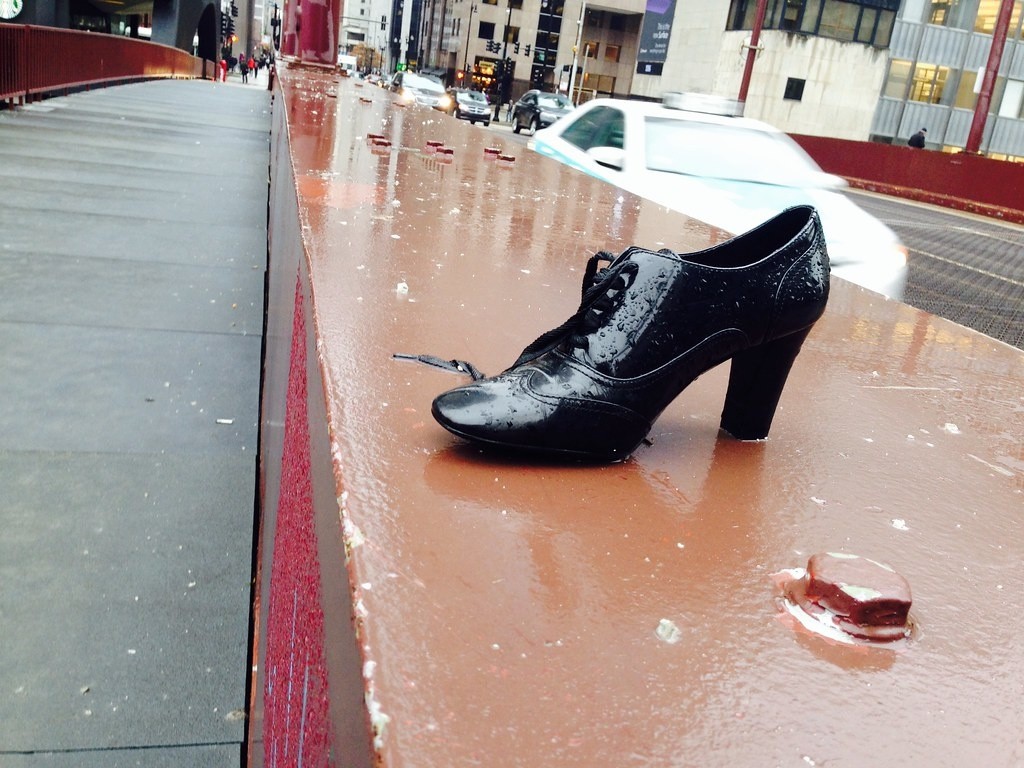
<box><xmin>394</xmin><ymin>205</ymin><xmax>831</xmax><ymax>462</ymax></box>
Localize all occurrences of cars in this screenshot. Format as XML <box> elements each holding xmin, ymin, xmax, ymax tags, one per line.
<box><xmin>526</xmin><ymin>90</ymin><xmax>909</xmax><ymax>305</ymax></box>
<box><xmin>348</xmin><ymin>70</ymin><xmax>491</xmax><ymax>127</ymax></box>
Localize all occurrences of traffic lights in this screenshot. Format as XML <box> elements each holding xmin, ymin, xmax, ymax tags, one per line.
<box><xmin>493</xmin><ymin>58</ymin><xmax>511</xmax><ymax>78</ymax></box>
<box><xmin>525</xmin><ymin>43</ymin><xmax>531</xmax><ymax>57</ymax></box>
<box><xmin>221</xmin><ymin>11</ymin><xmax>236</xmax><ymax>42</ymax></box>
<box><xmin>514</xmin><ymin>40</ymin><xmax>520</xmax><ymax>53</ymax></box>
<box><xmin>533</xmin><ymin>66</ymin><xmax>543</xmax><ymax>85</ymax></box>
<box><xmin>485</xmin><ymin>39</ymin><xmax>501</xmax><ymax>54</ymax></box>
<box><xmin>232</xmin><ymin>5</ymin><xmax>238</xmax><ymax>17</ymax></box>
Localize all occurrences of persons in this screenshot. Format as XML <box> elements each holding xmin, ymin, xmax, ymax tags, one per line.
<box><xmin>908</xmin><ymin>128</ymin><xmax>927</xmax><ymax>149</ymax></box>
<box><xmin>239</xmin><ymin>52</ymin><xmax>245</xmax><ymax>74</ymax></box>
<box><xmin>240</xmin><ymin>60</ymin><xmax>249</xmax><ymax>83</ymax></box>
<box><xmin>260</xmin><ymin>54</ymin><xmax>270</xmax><ymax>69</ymax></box>
<box><xmin>247</xmin><ymin>57</ymin><xmax>260</xmax><ymax>78</ymax></box>
<box><xmin>505</xmin><ymin>100</ymin><xmax>513</xmax><ymax>122</ymax></box>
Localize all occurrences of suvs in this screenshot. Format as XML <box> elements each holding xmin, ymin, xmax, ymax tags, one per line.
<box><xmin>510</xmin><ymin>89</ymin><xmax>577</xmax><ymax>136</ymax></box>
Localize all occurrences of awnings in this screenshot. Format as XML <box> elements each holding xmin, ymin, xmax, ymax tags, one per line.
<box><xmin>422</xmin><ymin>68</ymin><xmax>434</xmax><ymax>75</ymax></box>
<box><xmin>431</xmin><ymin>68</ymin><xmax>446</xmax><ymax>78</ymax></box>
